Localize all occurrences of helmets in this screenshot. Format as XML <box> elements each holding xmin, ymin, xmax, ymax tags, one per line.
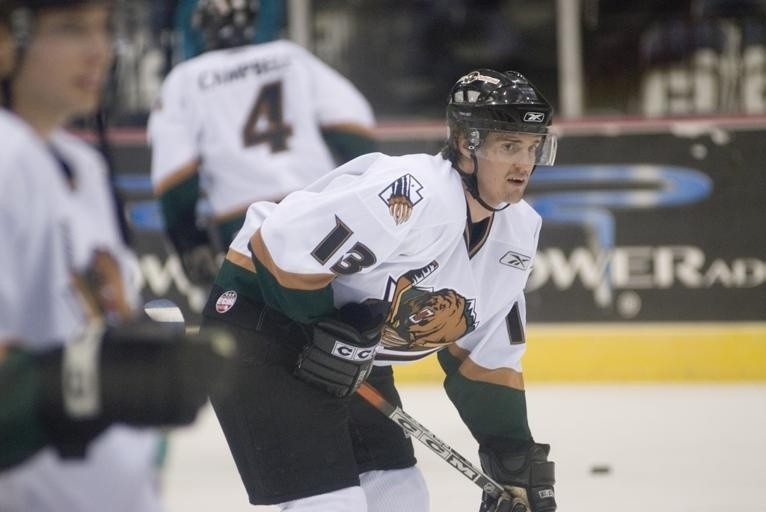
<box><xmin>446</xmin><ymin>68</ymin><xmax>558</xmax><ymax>167</ymax></box>
<box><xmin>191</xmin><ymin>0</ymin><xmax>260</xmax><ymax>47</ymax></box>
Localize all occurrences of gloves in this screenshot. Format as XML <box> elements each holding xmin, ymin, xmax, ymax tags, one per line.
<box><xmin>478</xmin><ymin>437</ymin><xmax>557</xmax><ymax>512</ymax></box>
<box><xmin>40</xmin><ymin>327</ymin><xmax>214</xmax><ymax>461</ymax></box>
<box><xmin>291</xmin><ymin>302</ymin><xmax>388</xmax><ymax>401</ymax></box>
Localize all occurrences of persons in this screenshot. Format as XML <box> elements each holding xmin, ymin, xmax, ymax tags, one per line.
<box><xmin>0</xmin><ymin>1</ymin><xmax>240</xmax><ymax>511</ymax></box>
<box><xmin>145</xmin><ymin>0</ymin><xmax>380</xmax><ymax>273</ymax></box>
<box><xmin>163</xmin><ymin>68</ymin><xmax>559</xmax><ymax>511</ymax></box>
